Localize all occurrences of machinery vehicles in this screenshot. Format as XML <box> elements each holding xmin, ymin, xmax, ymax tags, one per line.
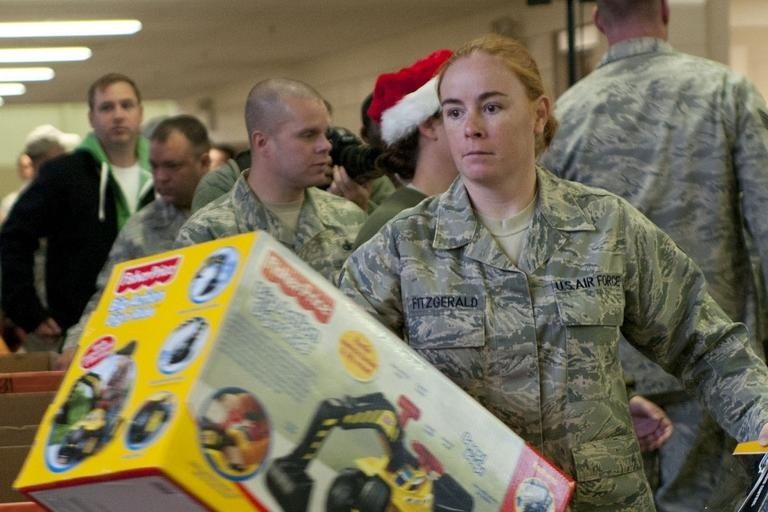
<box><xmin>266</xmin><ymin>392</ymin><xmax>475</xmax><ymax>512</ymax></box>
<box><xmin>52</xmin><ymin>370</ymin><xmax>109</xmax><ymax>465</ymax></box>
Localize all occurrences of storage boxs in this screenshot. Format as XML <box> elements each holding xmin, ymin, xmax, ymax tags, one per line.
<box><xmin>0</xmin><ymin>231</ymin><xmax>577</xmax><ymax>512</ymax></box>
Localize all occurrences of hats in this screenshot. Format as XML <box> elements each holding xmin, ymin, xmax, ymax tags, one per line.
<box><xmin>366</xmin><ymin>48</ymin><xmax>455</xmax><ymax>148</ymax></box>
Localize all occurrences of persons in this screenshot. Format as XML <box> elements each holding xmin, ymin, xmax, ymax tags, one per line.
<box><xmin>0</xmin><ymin>74</ymin><xmax>158</xmax><ymax>352</ymax></box>
<box><xmin>334</xmin><ymin>31</ymin><xmax>768</xmax><ymax>512</ymax></box>
<box><xmin>168</xmin><ymin>78</ymin><xmax>368</xmax><ymax>282</ymax></box>
<box><xmin>326</xmin><ymin>126</ymin><xmax>393</xmax><ymax>212</ymax></box>
<box><xmin>357</xmin><ymin>93</ymin><xmax>399</xmax><ymax>198</ymax></box>
<box><xmin>58</xmin><ymin>115</ymin><xmax>250</xmax><ymax>371</ymax></box>
<box><xmin>342</xmin><ymin>46</ymin><xmax>673</xmax><ymax>456</ymax></box>
<box><xmin>1</xmin><ymin>125</ymin><xmax>80</xmax><ymax>350</ymax></box>
<box><xmin>537</xmin><ymin>0</ymin><xmax>768</xmax><ymax>364</ymax></box>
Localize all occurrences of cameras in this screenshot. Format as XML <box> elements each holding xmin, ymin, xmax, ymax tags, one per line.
<box><xmin>325</xmin><ymin>126</ymin><xmax>386</xmax><ymax>186</ymax></box>
